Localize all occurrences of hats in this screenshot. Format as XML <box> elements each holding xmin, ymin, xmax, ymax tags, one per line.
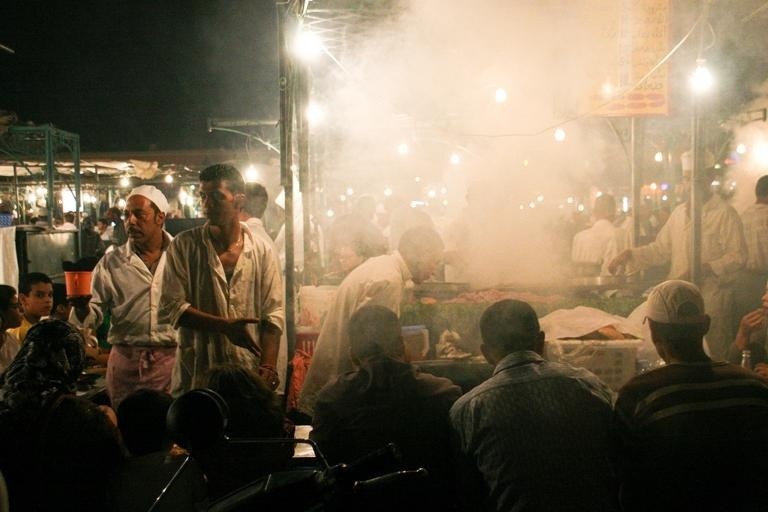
<box><xmin>681</xmin><ymin>148</ymin><xmax>714</xmax><ymax>171</ymax></box>
<box><xmin>127</xmin><ymin>184</ymin><xmax>168</xmax><ymax>217</ymax></box>
<box><xmin>639</xmin><ymin>280</ymin><xmax>704</xmax><ymax>325</ymax></box>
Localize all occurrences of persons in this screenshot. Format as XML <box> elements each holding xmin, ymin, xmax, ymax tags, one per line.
<box><xmin>1</xmin><ymin>161</ymin><xmax>767</xmax><ymax>512</ymax></box>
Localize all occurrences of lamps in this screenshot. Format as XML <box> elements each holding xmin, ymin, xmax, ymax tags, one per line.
<box><xmin>685</xmin><ymin>15</ymin><xmax>718</xmax><ymax>96</ymax></box>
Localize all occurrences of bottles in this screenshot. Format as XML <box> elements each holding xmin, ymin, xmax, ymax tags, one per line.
<box><xmin>19</xmin><ymin>209</ymin><xmax>27</xmax><ymax>225</ymax></box>
<box><xmin>739</xmin><ymin>349</ymin><xmax>753</xmax><ymax>370</ymax></box>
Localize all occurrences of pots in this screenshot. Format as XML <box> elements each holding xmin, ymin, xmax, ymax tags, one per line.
<box><xmin>569</xmin><ymin>268</ymin><xmax>642</xmax><ymax>286</ymax></box>
<box><xmin>567</xmin><ymin>257</ymin><xmax>604</xmax><ymax>279</ymax></box>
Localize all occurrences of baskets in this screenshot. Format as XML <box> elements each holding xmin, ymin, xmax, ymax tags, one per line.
<box><xmin>545</xmin><ymin>338</ymin><xmax>644</xmax><ymax>394</ymax></box>
